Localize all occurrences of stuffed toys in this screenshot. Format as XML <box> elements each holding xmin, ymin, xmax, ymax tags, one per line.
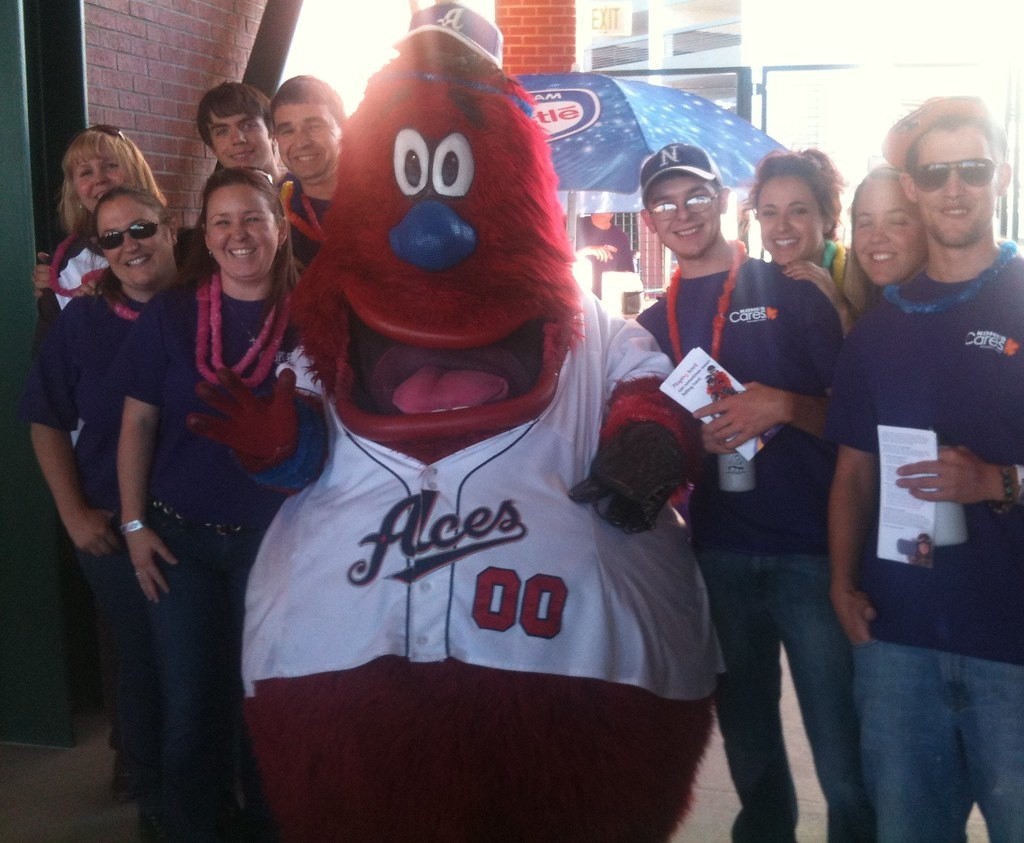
<box><xmin>240</xmin><ymin>2</ymin><xmax>727</xmax><ymax>843</ymax></box>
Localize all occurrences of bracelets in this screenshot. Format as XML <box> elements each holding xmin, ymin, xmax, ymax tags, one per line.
<box><xmin>119</xmin><ymin>520</ymin><xmax>144</xmax><ymax>535</ymax></box>
<box><xmin>988</xmin><ymin>464</ymin><xmax>1024</xmax><ymax>515</ymax></box>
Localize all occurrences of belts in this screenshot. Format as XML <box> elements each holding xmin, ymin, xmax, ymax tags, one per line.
<box><xmin>149</xmin><ymin>500</ymin><xmax>242</xmax><ymax>534</ymax></box>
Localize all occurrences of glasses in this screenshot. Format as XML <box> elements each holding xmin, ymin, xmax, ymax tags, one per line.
<box><xmin>646</xmin><ymin>190</ymin><xmax>720</xmax><ymax>221</ymax></box>
<box><xmin>65</xmin><ymin>125</ymin><xmax>129</xmax><ymax>144</ymax></box>
<box><xmin>93</xmin><ymin>220</ymin><xmax>166</xmax><ymax>252</ymax></box>
<box><xmin>906</xmin><ymin>157</ymin><xmax>1000</xmax><ymax>191</ymax></box>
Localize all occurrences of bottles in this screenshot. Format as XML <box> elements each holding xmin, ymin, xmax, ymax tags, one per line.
<box><xmin>718</xmin><ymin>451</ymin><xmax>756</xmax><ymax>490</ymax></box>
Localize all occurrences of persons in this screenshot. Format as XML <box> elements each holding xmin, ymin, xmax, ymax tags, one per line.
<box><xmin>116</xmin><ymin>165</ymin><xmax>299</xmax><ymax>843</ymax></box>
<box><xmin>275</xmin><ymin>74</ymin><xmax>348</xmax><ymax>271</ymax></box>
<box><xmin>33</xmin><ymin>123</ymin><xmax>194</xmax><ymax>374</ymax></box>
<box><xmin>634</xmin><ymin>143</ymin><xmax>873</xmax><ymax>843</ymax></box>
<box><xmin>20</xmin><ymin>187</ymin><xmax>178</xmax><ymax>806</ymax></box>
<box><xmin>32</xmin><ymin>82</ymin><xmax>286</xmax><ymax>299</ymax></box>
<box><xmin>748</xmin><ymin>148</ymin><xmax>928</xmax><ymax>339</ymax></box>
<box><xmin>823</xmin><ymin>95</ymin><xmax>1024</xmax><ymax>843</ymax></box>
<box><xmin>577</xmin><ymin>212</ymin><xmax>635</xmax><ymax>298</ymax></box>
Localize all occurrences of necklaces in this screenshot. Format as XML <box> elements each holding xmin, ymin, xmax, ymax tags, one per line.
<box><xmin>666</xmin><ymin>239</ymin><xmax>747</xmax><ymax>366</ymax></box>
<box><xmin>50</xmin><ymin>233</ymin><xmax>84</xmax><ymax>297</ymax></box>
<box><xmin>280</xmin><ymin>180</ymin><xmax>323</xmax><ymax>244</ymax></box>
<box><xmin>884</xmin><ymin>240</ymin><xmax>1018</xmax><ymax>315</ymax></box>
<box><xmin>104</xmin><ymin>292</ymin><xmax>140</xmax><ymax>321</ymax></box>
<box><xmin>196</xmin><ymin>273</ymin><xmax>289</xmax><ymax>387</ymax></box>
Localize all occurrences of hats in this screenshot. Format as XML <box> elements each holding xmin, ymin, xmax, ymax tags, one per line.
<box><xmin>881</xmin><ymin>96</ymin><xmax>996</xmax><ymax>166</ymax></box>
<box><xmin>639</xmin><ymin>143</ymin><xmax>724</xmax><ymax>204</ymax></box>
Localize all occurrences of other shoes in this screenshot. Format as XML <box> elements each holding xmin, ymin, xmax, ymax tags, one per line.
<box><xmin>109</xmin><ymin>758</ymin><xmax>150</xmax><ymax>800</ymax></box>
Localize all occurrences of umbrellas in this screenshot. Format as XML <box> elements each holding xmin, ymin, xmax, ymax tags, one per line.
<box><xmin>517</xmin><ymin>73</ymin><xmax>789</xmax><ymax>254</ymax></box>
